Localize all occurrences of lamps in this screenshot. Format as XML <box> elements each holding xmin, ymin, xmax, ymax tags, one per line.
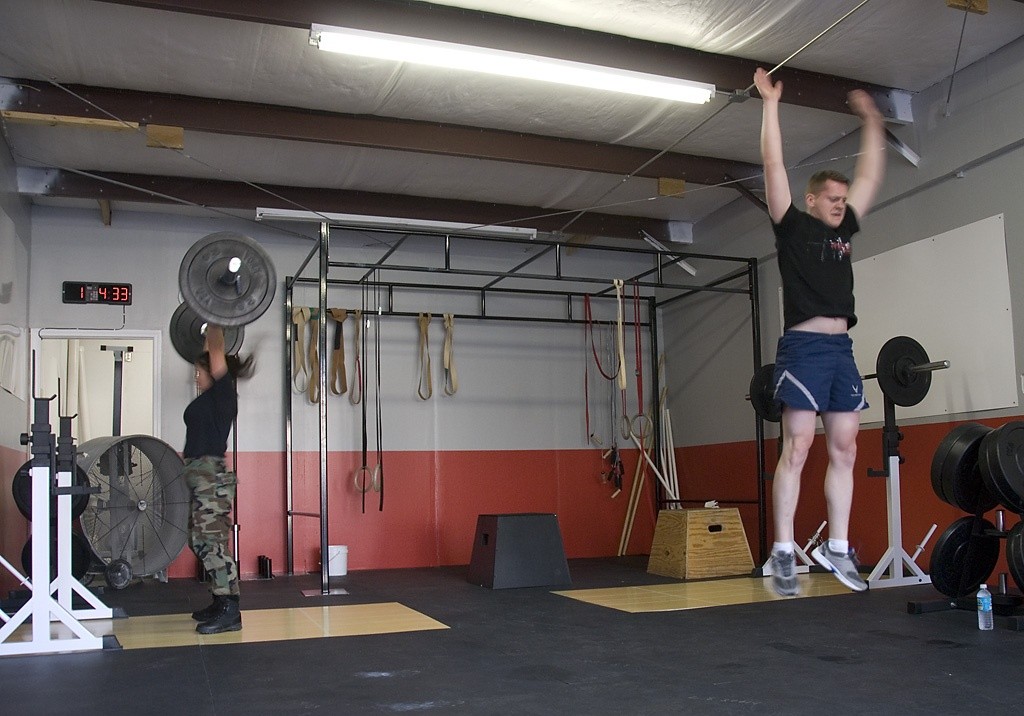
<box><xmin>308</xmin><ymin>23</ymin><xmax>716</xmax><ymax>104</ymax></box>
<box><xmin>255</xmin><ymin>207</ymin><xmax>538</xmax><ymax>241</ymax></box>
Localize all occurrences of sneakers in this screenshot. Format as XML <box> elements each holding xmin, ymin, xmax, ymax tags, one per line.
<box><xmin>772</xmin><ymin>551</ymin><xmax>800</xmax><ymax>595</ymax></box>
<box><xmin>811</xmin><ymin>540</ymin><xmax>867</xmax><ymax>591</ymax></box>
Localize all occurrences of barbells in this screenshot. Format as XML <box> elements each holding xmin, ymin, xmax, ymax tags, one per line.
<box><xmin>744</xmin><ymin>336</ymin><xmax>952</xmax><ymax>422</ymax></box>
<box><xmin>169</xmin><ymin>231</ymin><xmax>278</xmax><ymax>366</ymax></box>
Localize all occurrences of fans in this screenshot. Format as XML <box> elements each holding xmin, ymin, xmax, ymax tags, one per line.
<box><xmin>72</xmin><ymin>434</ymin><xmax>189</xmax><ymax>582</ymax></box>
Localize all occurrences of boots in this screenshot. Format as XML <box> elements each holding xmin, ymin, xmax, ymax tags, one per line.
<box><xmin>196</xmin><ymin>596</ymin><xmax>242</xmax><ymax>634</ymax></box>
<box><xmin>192</xmin><ymin>594</ymin><xmax>225</xmax><ymax>622</ymax></box>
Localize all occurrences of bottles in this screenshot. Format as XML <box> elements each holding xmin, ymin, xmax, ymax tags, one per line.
<box><xmin>976</xmin><ymin>583</ymin><xmax>994</xmax><ymax>631</ymax></box>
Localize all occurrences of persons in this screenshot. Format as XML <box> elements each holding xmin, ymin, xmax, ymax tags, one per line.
<box><xmin>753</xmin><ymin>68</ymin><xmax>885</xmax><ymax>596</ymax></box>
<box><xmin>182</xmin><ymin>323</ymin><xmax>259</xmax><ymax>634</ymax></box>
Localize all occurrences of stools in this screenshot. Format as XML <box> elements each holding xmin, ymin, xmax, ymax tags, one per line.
<box><xmin>468</xmin><ymin>514</ymin><xmax>576</xmax><ymax>592</ymax></box>
<box><xmin>646</xmin><ymin>508</ymin><xmax>756</xmax><ymax>578</ymax></box>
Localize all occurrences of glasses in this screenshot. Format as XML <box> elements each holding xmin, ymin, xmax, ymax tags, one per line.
<box><xmin>195</xmin><ymin>371</ymin><xmax>209</xmax><ymax>377</ymax></box>
